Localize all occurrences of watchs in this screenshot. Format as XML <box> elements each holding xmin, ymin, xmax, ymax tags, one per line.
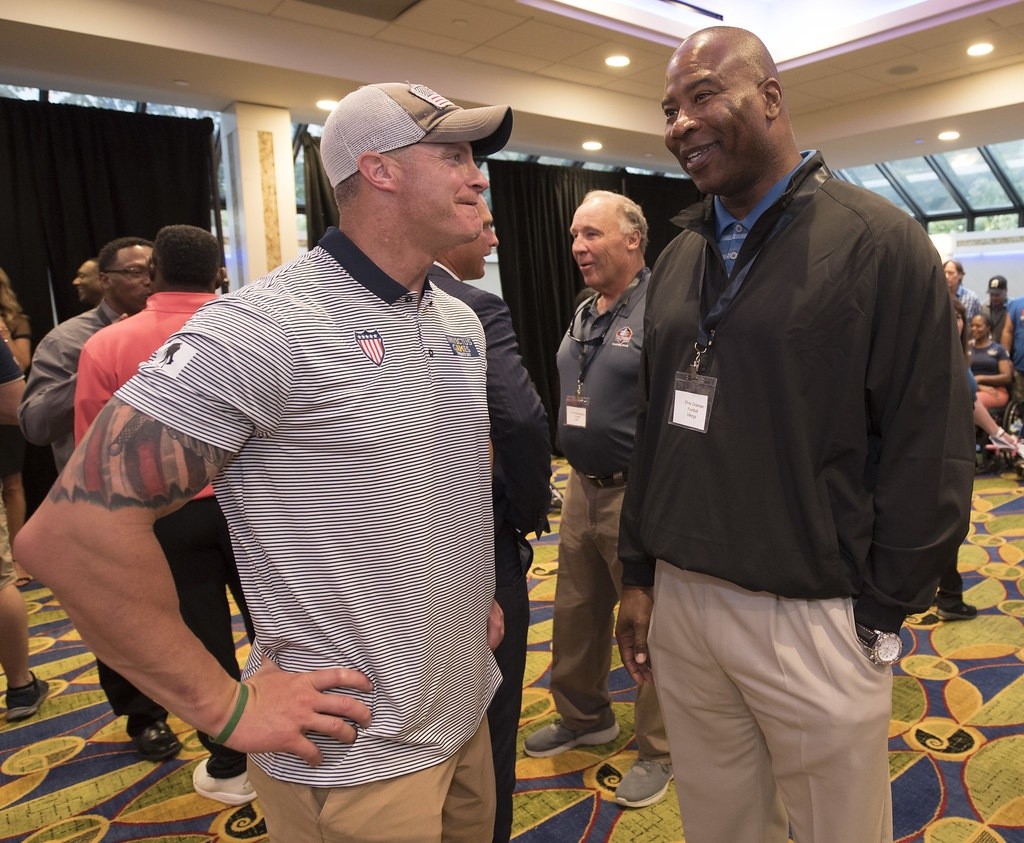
<box><xmin>854</xmin><ymin>622</ymin><xmax>905</xmax><ymax>668</ymax></box>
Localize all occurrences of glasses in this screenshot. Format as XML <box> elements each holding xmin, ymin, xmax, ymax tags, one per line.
<box><xmin>102</xmin><ymin>266</ymin><xmax>156</xmax><ymax>282</ymax></box>
<box><xmin>568</xmin><ymin>297</ymin><xmax>631</xmax><ymax>347</ymax></box>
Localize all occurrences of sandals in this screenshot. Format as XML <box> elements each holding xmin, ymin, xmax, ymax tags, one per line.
<box><xmin>11</xmin><ymin>558</ymin><xmax>29</xmax><ymax>586</ymax></box>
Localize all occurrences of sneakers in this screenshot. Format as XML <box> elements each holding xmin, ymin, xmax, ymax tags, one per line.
<box><xmin>5</xmin><ymin>671</ymin><xmax>49</xmax><ymax>719</ymax></box>
<box><xmin>524</xmin><ymin>716</ymin><xmax>620</xmax><ymax>757</ymax></box>
<box><xmin>615</xmin><ymin>757</ymin><xmax>673</xmax><ymax>807</ymax></box>
<box><xmin>192</xmin><ymin>758</ymin><xmax>258</xmax><ymax>805</ymax></box>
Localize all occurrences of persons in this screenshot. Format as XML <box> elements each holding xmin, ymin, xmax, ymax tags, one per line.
<box><xmin>616</xmin><ymin>27</ymin><xmax>976</xmax><ymax>843</ymax></box>
<box><xmin>12</xmin><ymin>83</ymin><xmax>514</xmax><ymax>843</ymax></box>
<box><xmin>0</xmin><ymin>225</ymin><xmax>259</xmax><ymax>807</ymax></box>
<box><xmin>934</xmin><ymin>259</ymin><xmax>1024</xmax><ymax>622</ymax></box>
<box><xmin>524</xmin><ymin>190</ymin><xmax>676</xmax><ymax>809</ymax></box>
<box><xmin>425</xmin><ymin>190</ymin><xmax>552</xmax><ymax>843</ymax></box>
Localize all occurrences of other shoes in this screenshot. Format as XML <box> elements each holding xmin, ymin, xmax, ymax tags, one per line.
<box><xmin>1011</xmin><ymin>438</ymin><xmax>1021</xmax><ymax>457</ymax></box>
<box><xmin>938</xmin><ymin>603</ymin><xmax>978</xmax><ymax>620</ymax></box>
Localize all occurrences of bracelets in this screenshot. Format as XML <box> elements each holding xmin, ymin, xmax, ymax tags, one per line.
<box><xmin>995</xmin><ymin>426</ymin><xmax>1004</xmax><ymax>438</ymax></box>
<box><xmin>212</xmin><ymin>682</ymin><xmax>249</xmax><ymax>746</ymax></box>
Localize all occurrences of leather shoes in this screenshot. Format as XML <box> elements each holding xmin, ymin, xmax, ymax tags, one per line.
<box><xmin>132</xmin><ymin>719</ymin><xmax>184</xmax><ymax>762</ymax></box>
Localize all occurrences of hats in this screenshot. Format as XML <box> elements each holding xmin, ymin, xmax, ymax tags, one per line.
<box><xmin>985</xmin><ymin>275</ymin><xmax>1006</xmax><ymax>295</ymax></box>
<box><xmin>320</xmin><ymin>81</ymin><xmax>513</xmax><ymax>188</ymax></box>
